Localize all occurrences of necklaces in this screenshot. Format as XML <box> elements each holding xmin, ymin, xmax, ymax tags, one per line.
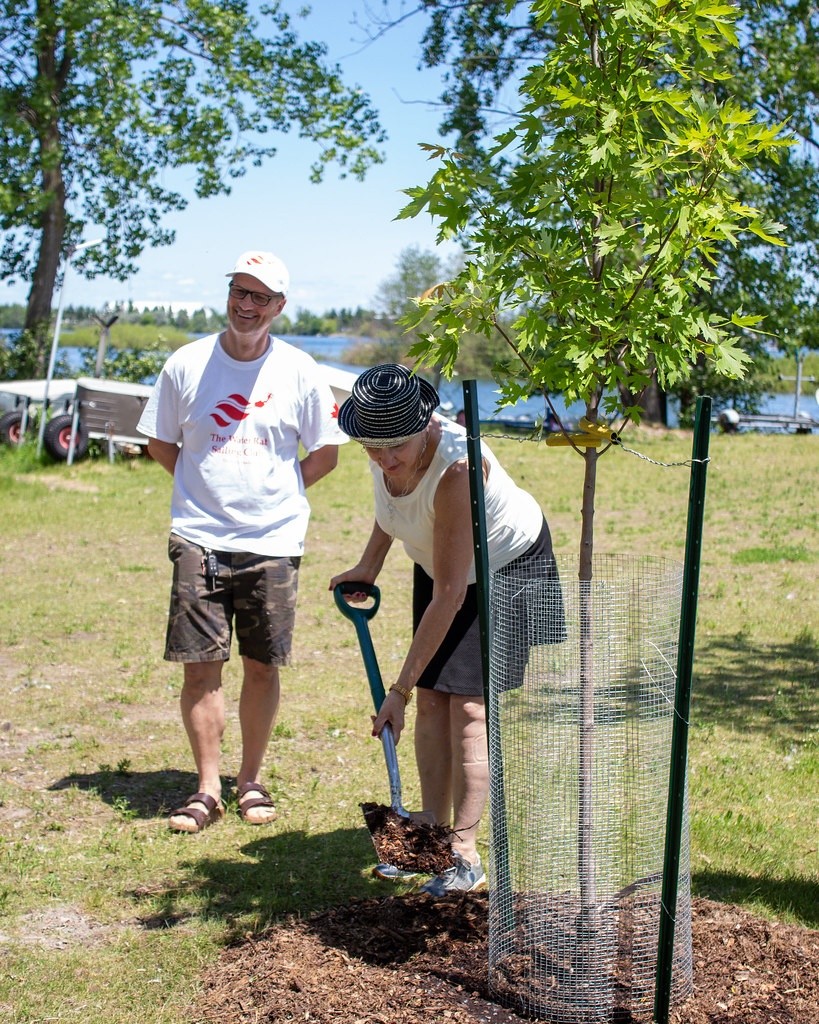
<box><xmin>386</xmin><ymin>431</ymin><xmax>433</xmax><ymax>543</ymax></box>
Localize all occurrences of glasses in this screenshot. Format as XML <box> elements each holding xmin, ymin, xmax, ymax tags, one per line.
<box><xmin>228</xmin><ymin>279</ymin><xmax>284</xmax><ymax>306</ymax></box>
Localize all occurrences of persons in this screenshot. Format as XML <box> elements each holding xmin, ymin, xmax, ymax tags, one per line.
<box><xmin>136</xmin><ymin>249</ymin><xmax>351</xmax><ymax>832</ymax></box>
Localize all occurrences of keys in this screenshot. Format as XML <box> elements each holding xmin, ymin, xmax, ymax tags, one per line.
<box><xmin>201</xmin><ymin>555</ymin><xmax>207</xmax><ymax>576</ymax></box>
<box><xmin>205</xmin><ymin>554</ymin><xmax>219</xmax><ymax>590</ymax></box>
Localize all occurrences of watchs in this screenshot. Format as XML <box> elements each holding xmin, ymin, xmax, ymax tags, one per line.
<box><xmin>389</xmin><ymin>683</ymin><xmax>413</xmax><ymax>705</ymax></box>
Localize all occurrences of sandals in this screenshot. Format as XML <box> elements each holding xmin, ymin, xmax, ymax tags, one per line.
<box><xmin>167</xmin><ymin>783</ymin><xmax>277</xmax><ymax>833</ymax></box>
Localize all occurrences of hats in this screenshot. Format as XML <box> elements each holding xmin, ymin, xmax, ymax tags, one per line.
<box><xmin>224</xmin><ymin>250</ymin><xmax>289</xmax><ymax>299</ymax></box>
<box><xmin>337</xmin><ymin>362</ymin><xmax>441</xmax><ymax>450</ymax></box>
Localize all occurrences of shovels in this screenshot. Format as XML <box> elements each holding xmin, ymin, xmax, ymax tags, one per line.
<box><xmin>331</xmin><ymin>578</ymin><xmax>459</xmax><ymax>876</ymax></box>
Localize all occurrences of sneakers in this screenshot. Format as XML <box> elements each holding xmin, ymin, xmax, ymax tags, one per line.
<box><xmin>371</xmin><ymin>846</ymin><xmax>488</xmax><ymax>897</ymax></box>
